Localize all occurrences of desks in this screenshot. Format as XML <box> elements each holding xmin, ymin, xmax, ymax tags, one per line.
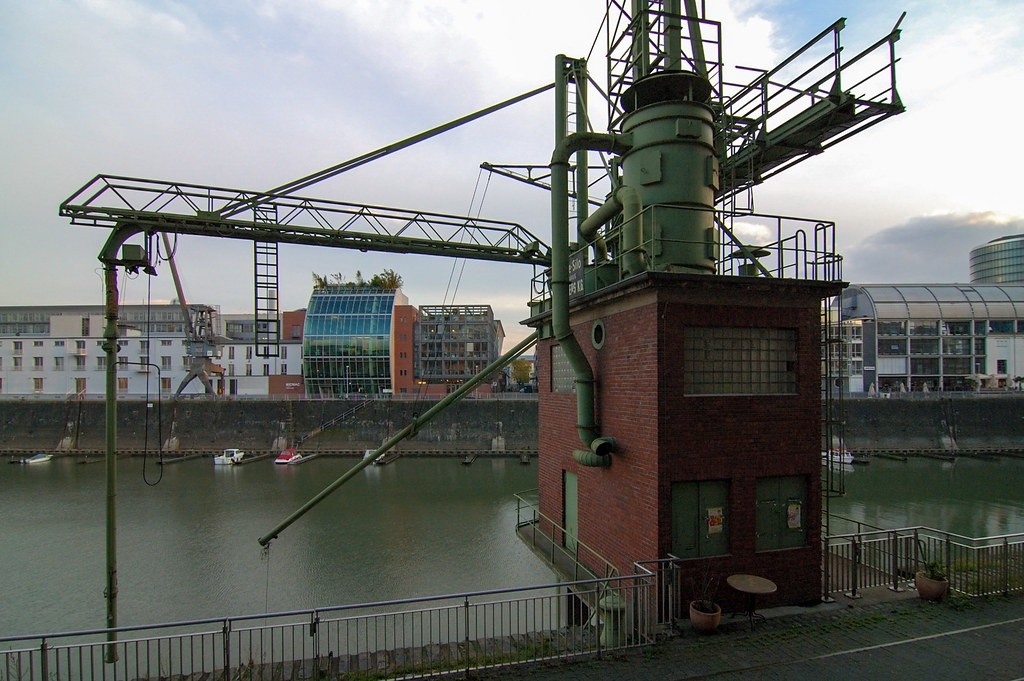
<box><xmin>726</xmin><ymin>575</ymin><xmax>778</xmax><ymax>633</ymax></box>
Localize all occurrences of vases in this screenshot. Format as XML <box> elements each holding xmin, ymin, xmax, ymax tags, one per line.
<box><xmin>688</xmin><ymin>599</ymin><xmax>722</xmax><ymax>634</ymax></box>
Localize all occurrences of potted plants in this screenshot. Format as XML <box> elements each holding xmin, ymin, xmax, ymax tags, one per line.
<box><xmin>914</xmin><ymin>559</ymin><xmax>949</xmax><ymax>601</ymax></box>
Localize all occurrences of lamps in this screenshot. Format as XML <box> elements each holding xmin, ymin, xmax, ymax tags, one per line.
<box><xmin>144</xmin><ymin>266</ymin><xmax>158</xmax><ymax>277</ymax></box>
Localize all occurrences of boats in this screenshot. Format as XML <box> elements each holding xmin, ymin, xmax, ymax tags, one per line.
<box><xmin>362</xmin><ymin>449</ymin><xmax>385</xmax><ymax>465</ymax></box>
<box><xmin>19</xmin><ymin>453</ymin><xmax>53</xmax><ymax>464</ymax></box>
<box><xmin>825</xmin><ymin>447</ymin><xmax>855</xmax><ymax>464</ymax></box>
<box><xmin>214</xmin><ymin>448</ymin><xmax>245</xmax><ymax>465</ymax></box>
<box><xmin>274</xmin><ymin>448</ymin><xmax>302</xmax><ymax>464</ymax></box>
